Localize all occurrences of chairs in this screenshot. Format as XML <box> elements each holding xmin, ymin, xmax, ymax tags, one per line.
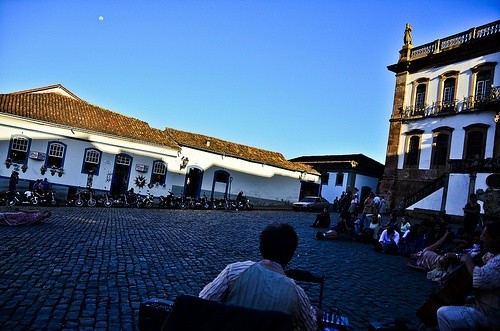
<box><xmin>283</xmin><ymin>269</ymin><xmax>325</xmax><ymax>310</ymax></box>
<box><xmin>162</xmin><ymin>294</ymin><xmax>295</xmax><ymax>331</ymax></box>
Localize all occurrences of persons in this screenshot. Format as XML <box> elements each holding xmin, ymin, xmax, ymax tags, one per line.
<box><xmin>9</xmin><ymin>171</ymin><xmax>19</xmax><ymax>191</ymax></box>
<box><xmin>0</xmin><ymin>209</ymin><xmax>51</xmax><ymax>226</ymax></box>
<box><xmin>309</xmin><ymin>205</ymin><xmax>331</xmax><ymax>228</ymax></box>
<box><xmin>235</xmin><ymin>191</ymin><xmax>248</xmax><ymax>211</ymax></box>
<box><xmin>198</xmin><ymin>224</ymin><xmax>323</xmax><ymax>331</ymax></box>
<box><xmin>437</xmin><ymin>220</ymin><xmax>500</xmax><ymax>331</ymax></box>
<box><xmin>33</xmin><ymin>178</ymin><xmax>50</xmax><ymax>190</ymax></box>
<box><xmin>334</xmin><ymin>184</ymin><xmax>413</xmax><ymax>252</ymax></box>
<box><xmin>464</xmin><ymin>193</ymin><xmax>480</xmax><ymax>231</ymax></box>
<box><xmin>406</xmin><ymin>219</ymin><xmax>456</xmax><ymax>271</ymax></box>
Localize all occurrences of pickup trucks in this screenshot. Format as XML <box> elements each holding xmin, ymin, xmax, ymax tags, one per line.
<box><xmin>293</xmin><ymin>196</ymin><xmax>331</xmax><ymax>211</ymax></box>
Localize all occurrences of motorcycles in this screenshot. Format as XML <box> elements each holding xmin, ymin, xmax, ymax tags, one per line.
<box><xmin>66</xmin><ymin>187</ymin><xmax>154</xmax><ymax>207</ymax></box>
<box><xmin>0</xmin><ymin>190</ymin><xmax>58</xmax><ymax>207</ymax></box>
<box><xmin>158</xmin><ymin>190</ymin><xmax>254</xmax><ymax>211</ymax></box>
